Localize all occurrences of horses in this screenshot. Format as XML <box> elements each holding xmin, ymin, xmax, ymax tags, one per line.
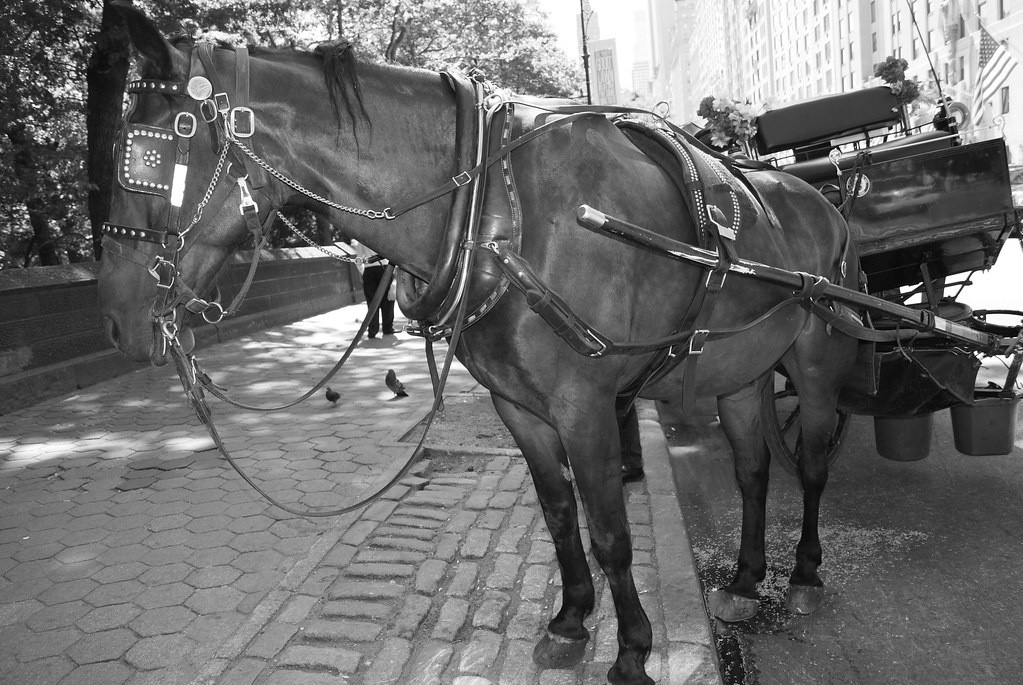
<box><xmin>86</xmin><ymin>0</ymin><xmax>861</xmax><ymax>685</ymax></box>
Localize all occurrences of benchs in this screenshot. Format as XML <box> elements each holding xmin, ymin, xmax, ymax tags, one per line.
<box><xmin>694</xmin><ymin>84</ymin><xmax>953</xmax><ymax>185</ymax></box>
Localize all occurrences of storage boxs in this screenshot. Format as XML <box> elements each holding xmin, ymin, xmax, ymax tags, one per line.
<box><xmin>951</xmin><ymin>388</ymin><xmax>1019</xmax><ymax>456</ymax></box>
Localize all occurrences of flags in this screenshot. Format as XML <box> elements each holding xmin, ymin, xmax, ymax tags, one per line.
<box><xmin>974</xmin><ymin>26</ymin><xmax>1018</xmax><ymax>126</ymax></box>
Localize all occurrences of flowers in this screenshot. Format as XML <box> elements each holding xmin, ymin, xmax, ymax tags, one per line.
<box><xmin>872</xmin><ymin>55</ymin><xmax>920</xmax><ymax>106</ymax></box>
<box><xmin>696</xmin><ymin>95</ymin><xmax>758</xmax><ymax>150</ymax></box>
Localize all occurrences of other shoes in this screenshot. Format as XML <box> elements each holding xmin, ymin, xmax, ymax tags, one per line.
<box><xmin>368</xmin><ymin>329</ymin><xmax>378</xmax><ymax>337</ymax></box>
<box><xmin>620</xmin><ymin>463</ymin><xmax>644</xmax><ymax>481</ymax></box>
<box><xmin>391</xmin><ymin>328</ymin><xmax>402</xmax><ymax>333</ymax></box>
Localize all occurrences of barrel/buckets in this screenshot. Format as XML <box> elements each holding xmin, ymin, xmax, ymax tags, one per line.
<box><xmin>949</xmin><ymin>385</ymin><xmax>1022</xmax><ymax>456</ymax></box>
<box><xmin>873</xmin><ymin>411</ymin><xmax>934</xmax><ymax>462</ymax></box>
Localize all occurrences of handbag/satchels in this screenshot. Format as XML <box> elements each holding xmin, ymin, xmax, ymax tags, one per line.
<box><xmin>388</xmin><ymin>278</ymin><xmax>397</xmax><ymax>301</ymax></box>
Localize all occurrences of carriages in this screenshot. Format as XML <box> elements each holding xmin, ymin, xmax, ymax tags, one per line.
<box><xmin>84</xmin><ymin>0</ymin><xmax>1023</xmax><ymax>685</ymax></box>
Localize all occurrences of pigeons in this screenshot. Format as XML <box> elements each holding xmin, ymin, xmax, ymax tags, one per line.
<box><xmin>384</xmin><ymin>369</ymin><xmax>408</xmax><ymax>396</ymax></box>
<box><xmin>326</xmin><ymin>387</ymin><xmax>341</xmax><ymax>404</ymax></box>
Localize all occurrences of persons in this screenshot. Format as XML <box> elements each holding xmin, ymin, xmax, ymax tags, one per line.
<box><xmin>349</xmin><ymin>237</ymin><xmax>401</xmax><ymax>339</ymax></box>
